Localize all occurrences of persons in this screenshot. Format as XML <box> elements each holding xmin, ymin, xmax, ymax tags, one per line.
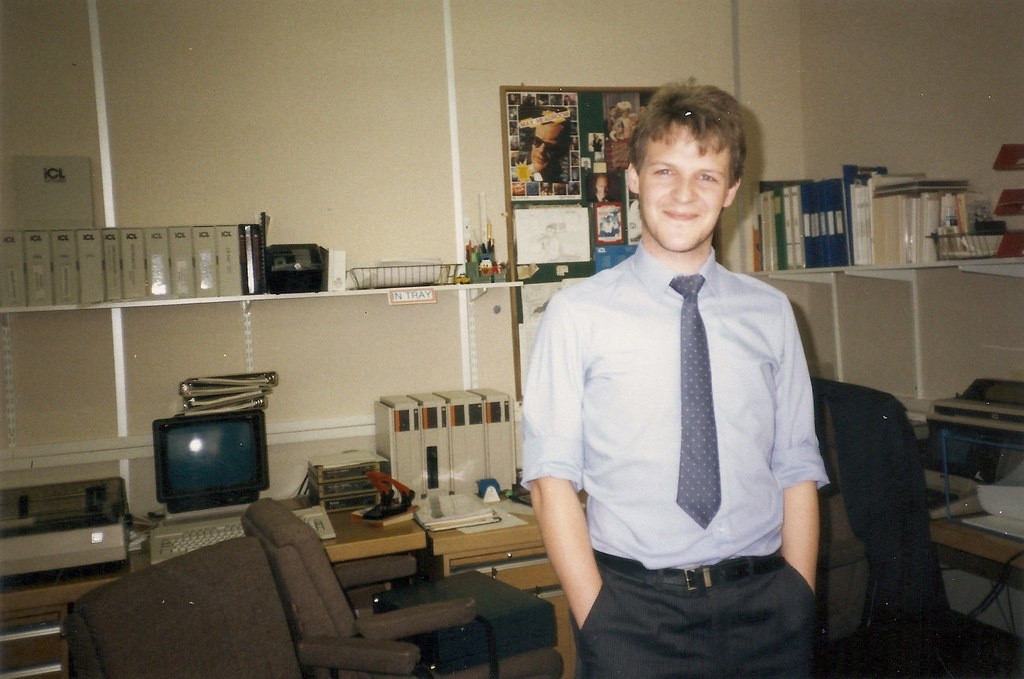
<box><xmin>605</xmin><ymin>101</ymin><xmax>646</xmax><ymax>139</ymax></box>
<box><xmin>590</xmin><ymin>133</ymin><xmax>603</xmax><ymax>151</ymax></box>
<box><xmin>590</xmin><ymin>175</ymin><xmax>615</xmax><ymax>204</ymax></box>
<box><xmin>581</xmin><ymin>161</ymin><xmax>591</xmax><ymax>172</ymax></box>
<box><xmin>599</xmin><ymin>214</ymin><xmax>619</xmax><ymax>237</ymax></box>
<box><xmin>526</xmin><ymin>124</ymin><xmax>566</xmax><ymax>183</ymax></box>
<box><xmin>522</xmin><ymin>83</ymin><xmax>829</xmax><ymax>679</ymax></box>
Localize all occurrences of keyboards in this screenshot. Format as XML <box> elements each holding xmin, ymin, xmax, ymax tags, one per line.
<box><xmin>149</xmin><ymin>506</ymin><xmax>335</xmax><ymax>567</ymax></box>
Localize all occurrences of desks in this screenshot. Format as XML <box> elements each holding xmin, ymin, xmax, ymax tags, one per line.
<box><xmin>0</xmin><ymin>496</ymin><xmax>427</xmax><ymax>678</ymax></box>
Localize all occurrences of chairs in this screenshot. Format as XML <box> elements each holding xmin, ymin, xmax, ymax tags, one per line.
<box><xmin>240</xmin><ymin>497</ymin><xmax>565</xmax><ymax>678</ymax></box>
<box><xmin>59</xmin><ymin>535</ymin><xmax>433</xmax><ymax>679</ymax></box>
<box><xmin>811</xmin><ymin>374</ymin><xmax>928</xmax><ymax>679</ymax></box>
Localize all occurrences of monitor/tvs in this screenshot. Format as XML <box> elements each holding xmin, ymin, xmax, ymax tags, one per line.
<box><xmin>151</xmin><ymin>409</ymin><xmax>271</xmax><ymax>515</ymax></box>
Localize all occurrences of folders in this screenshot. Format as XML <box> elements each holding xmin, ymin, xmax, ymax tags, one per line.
<box><xmin>757</xmin><ymin>179</ymin><xmax>850</xmax><ymax>274</ymax></box>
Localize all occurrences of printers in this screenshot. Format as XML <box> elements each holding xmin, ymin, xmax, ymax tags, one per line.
<box><xmin>920</xmin><ymin>378</ymin><xmax>1024</xmax><ymax>520</ymax></box>
<box><xmin>0</xmin><ymin>476</ymin><xmax>131</xmax><ymax>591</ymax></box>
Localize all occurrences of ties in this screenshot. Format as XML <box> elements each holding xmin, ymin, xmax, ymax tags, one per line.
<box><xmin>669</xmin><ymin>273</ymin><xmax>723</xmax><ymax>531</ymax></box>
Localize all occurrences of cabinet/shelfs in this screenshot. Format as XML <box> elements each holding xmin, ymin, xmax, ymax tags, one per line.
<box><xmin>412</xmin><ymin>481</ymin><xmax>588</xmax><ymax>678</ymax></box>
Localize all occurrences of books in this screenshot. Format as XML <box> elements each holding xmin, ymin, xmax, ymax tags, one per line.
<box><xmin>962</xmin><ymin>484</ymin><xmax>1024</xmax><ymax>538</ymax></box>
<box><xmin>752</xmin><ymin>166</ymin><xmax>987</xmax><ymax>273</ymax></box>
<box><xmin>179</xmin><ymin>372</ymin><xmax>278</xmax><ymax>415</ymax></box>
<box><xmin>353</xmin><ymin>493</ymin><xmax>502</xmax><ymax>531</ymax></box>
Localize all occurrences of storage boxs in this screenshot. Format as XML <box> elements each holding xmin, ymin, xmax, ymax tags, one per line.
<box><xmin>0</xmin><ymin>155</ymin><xmax>241</xmax><ymax>307</ymax></box>
<box><xmin>369</xmin><ymin>570</ymin><xmax>557</xmax><ymax>675</ymax></box>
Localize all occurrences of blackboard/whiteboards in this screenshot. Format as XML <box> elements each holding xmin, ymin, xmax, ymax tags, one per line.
<box><xmin>496</xmin><ymin>83</ymin><xmax>723</xmax><ymax>325</ymax></box>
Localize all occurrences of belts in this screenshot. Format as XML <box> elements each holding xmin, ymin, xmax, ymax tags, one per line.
<box><xmin>592</xmin><ymin>548</ymin><xmax>786</xmax><ymax>592</ymax></box>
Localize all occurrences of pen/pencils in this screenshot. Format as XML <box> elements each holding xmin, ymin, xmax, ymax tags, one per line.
<box><xmin>464</xmin><ymin>222</ymin><xmax>494</xmax><ymax>254</ymax></box>
